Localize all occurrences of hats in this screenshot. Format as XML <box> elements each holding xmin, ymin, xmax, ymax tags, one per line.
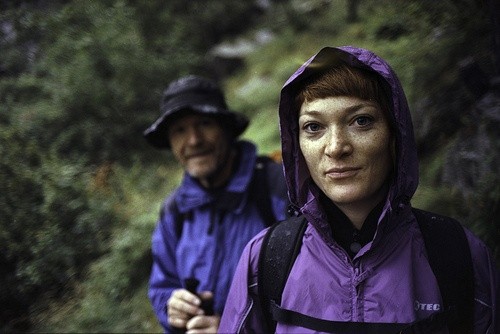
<box><xmin>143</xmin><ymin>75</ymin><xmax>248</xmax><ymax>152</ymax></box>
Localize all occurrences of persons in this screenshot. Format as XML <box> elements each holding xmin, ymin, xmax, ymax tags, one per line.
<box><xmin>144</xmin><ymin>77</ymin><xmax>301</xmax><ymax>334</ymax></box>
<box><xmin>217</xmin><ymin>46</ymin><xmax>499</xmax><ymax>334</ymax></box>
